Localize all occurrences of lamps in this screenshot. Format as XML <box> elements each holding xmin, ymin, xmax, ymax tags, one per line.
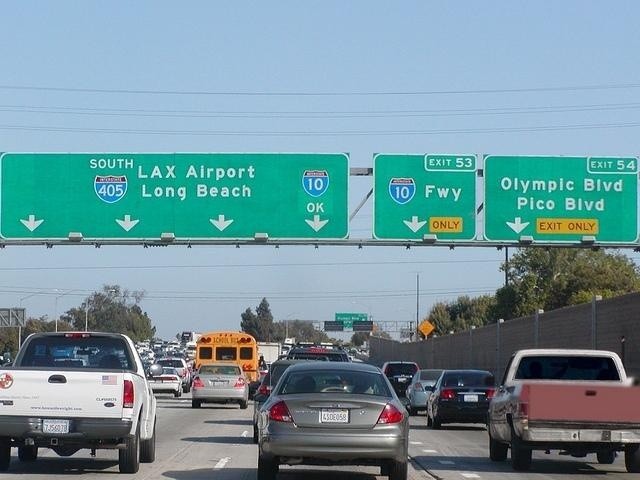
<box><xmin>422</xmin><ymin>234</ymin><xmax>437</xmax><ymax>243</ymax></box>
<box><xmin>518</xmin><ymin>236</ymin><xmax>533</xmax><ymax>244</ymax></box>
<box><xmin>160</xmin><ymin>232</ymin><xmax>175</xmax><ymax>242</ymax></box>
<box><xmin>254</xmin><ymin>233</ymin><xmax>269</xmax><ymax>242</ymax></box>
<box><xmin>580</xmin><ymin>235</ymin><xmax>596</xmax><ymax>242</ymax></box>
<box><xmin>68</xmin><ymin>232</ymin><xmax>83</xmax><ymax>241</ymax></box>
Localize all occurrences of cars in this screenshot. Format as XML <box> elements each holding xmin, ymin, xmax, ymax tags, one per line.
<box><xmin>253</xmin><ymin>341</ymin><xmax>420</xmax><ymax>479</ymax></box>
<box><xmin>52</xmin><ymin>331</ymin><xmax>200</xmax><ymax>398</ymax></box>
<box><xmin>404</xmin><ymin>368</ymin><xmax>445</xmax><ymax>416</ymax></box>
<box><xmin>425</xmin><ymin>368</ymin><xmax>497</xmax><ymax>429</ymax></box>
<box><xmin>191</xmin><ymin>362</ymin><xmax>250</xmax><ymax>409</ymax></box>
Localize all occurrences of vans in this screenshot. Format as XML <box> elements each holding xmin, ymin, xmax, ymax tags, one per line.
<box><xmin>196</xmin><ymin>331</ymin><xmax>260</xmax><ymax>393</ymax></box>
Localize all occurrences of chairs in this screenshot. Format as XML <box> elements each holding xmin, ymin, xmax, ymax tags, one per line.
<box><xmin>99</xmin><ymin>355</ymin><xmax>123</xmax><ymax>369</ymax></box>
<box><xmin>294</xmin><ymin>377</ymin><xmax>320</xmax><ymax>392</ymax></box>
<box><xmin>445</xmin><ymin>377</ymin><xmax>458</xmax><ymax>387</ymax></box>
<box><xmin>350</xmin><ymin>384</ymin><xmax>373</xmax><ymax>394</ymax></box>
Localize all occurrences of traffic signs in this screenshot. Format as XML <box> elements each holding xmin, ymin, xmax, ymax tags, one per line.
<box><xmin>335</xmin><ymin>312</ymin><xmax>368</xmax><ymax>329</ymax></box>
<box><xmin>373</xmin><ymin>153</ymin><xmax>478</xmax><ymax>243</ymax></box>
<box><xmin>480</xmin><ymin>154</ymin><xmax>639</xmax><ymax>246</ymax></box>
<box><xmin>2</xmin><ymin>153</ymin><xmax>352</xmax><ymax>245</ymax></box>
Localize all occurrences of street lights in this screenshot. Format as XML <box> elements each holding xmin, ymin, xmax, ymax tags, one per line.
<box><xmin>17</xmin><ymin>288</ymin><xmax>63</xmax><ymax>355</ymax></box>
<box><xmin>84</xmin><ymin>289</ymin><xmax>116</xmax><ymax>334</ymax></box>
<box><xmin>54</xmin><ymin>287</ymin><xmax>94</xmax><ymax>333</ymax></box>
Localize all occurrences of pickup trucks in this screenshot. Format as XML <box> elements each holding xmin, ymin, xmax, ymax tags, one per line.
<box><xmin>486</xmin><ymin>346</ymin><xmax>640</xmax><ymax>472</ymax></box>
<box><xmin>0</xmin><ymin>330</ymin><xmax>157</xmax><ymax>474</ymax></box>
